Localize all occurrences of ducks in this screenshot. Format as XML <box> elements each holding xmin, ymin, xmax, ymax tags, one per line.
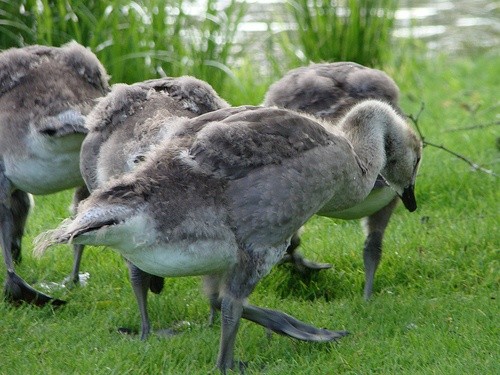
<box><xmin>78</xmin><ymin>56</ymin><xmax>402</xmax><ymax>342</ymax></box>
<box><xmin>31</xmin><ymin>97</ymin><xmax>424</xmax><ymax>375</ymax></box>
<box><xmin>0</xmin><ymin>36</ymin><xmax>114</xmax><ymax>309</ymax></box>
